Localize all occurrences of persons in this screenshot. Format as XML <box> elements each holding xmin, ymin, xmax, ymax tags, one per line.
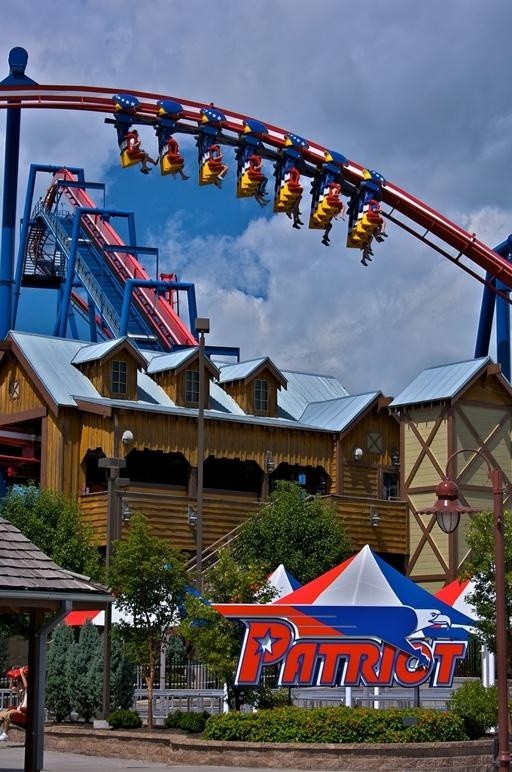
<box><xmin>0</xmin><ymin>664</ymin><xmax>28</xmax><ymax>742</ymax></box>
<box><xmin>119</xmin><ymin>129</ymin><xmax>388</xmax><ymax>268</ymax></box>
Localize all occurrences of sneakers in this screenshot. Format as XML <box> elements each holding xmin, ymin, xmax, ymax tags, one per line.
<box><xmin>359</xmin><ymin>232</ymin><xmax>389</xmax><ymax>266</ymax></box>
<box><xmin>140</xmin><ymin>156</ymin><xmax>190</xmax><ymax>180</ymax></box>
<box><xmin>215</xmin><ymin>175</ymin><xmax>224</xmax><ymax>190</ymax></box>
<box><xmin>0</xmin><ymin>733</ymin><xmax>9</xmax><ymax>742</ymax></box>
<box><xmin>259</xmin><ymin>191</ymin><xmax>272</xmax><ymax>209</ymax></box>
<box><xmin>321</xmin><ymin>215</ymin><xmax>345</xmax><ymax>246</ymax></box>
<box><xmin>291</xmin><ymin>219</ymin><xmax>305</xmax><ymax>232</ymax></box>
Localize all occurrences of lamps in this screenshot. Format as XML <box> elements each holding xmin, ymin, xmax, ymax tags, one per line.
<box><xmin>391</xmin><ymin>445</ymin><xmax>399</xmax><ymax>466</ymax></box>
<box><xmin>188</xmin><ymin>503</ymin><xmax>198</xmax><ymax>526</ymax></box>
<box><xmin>267</xmin><ymin>456</ymin><xmax>274</xmax><ymax>473</ymax></box>
<box><xmin>370</xmin><ymin>505</ymin><xmax>381</xmax><ymax>527</ymax></box>
<box><xmin>121</xmin><ymin>504</ymin><xmax>132</xmax><ymax>521</ymax></box>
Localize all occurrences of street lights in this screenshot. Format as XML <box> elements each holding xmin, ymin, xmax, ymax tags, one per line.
<box><xmin>194</xmin><ymin>317</ymin><xmax>210</xmax><ymax>591</ymax></box>
<box><xmin>415</xmin><ymin>449</ymin><xmax>511</xmax><ymax>772</ymax></box>
